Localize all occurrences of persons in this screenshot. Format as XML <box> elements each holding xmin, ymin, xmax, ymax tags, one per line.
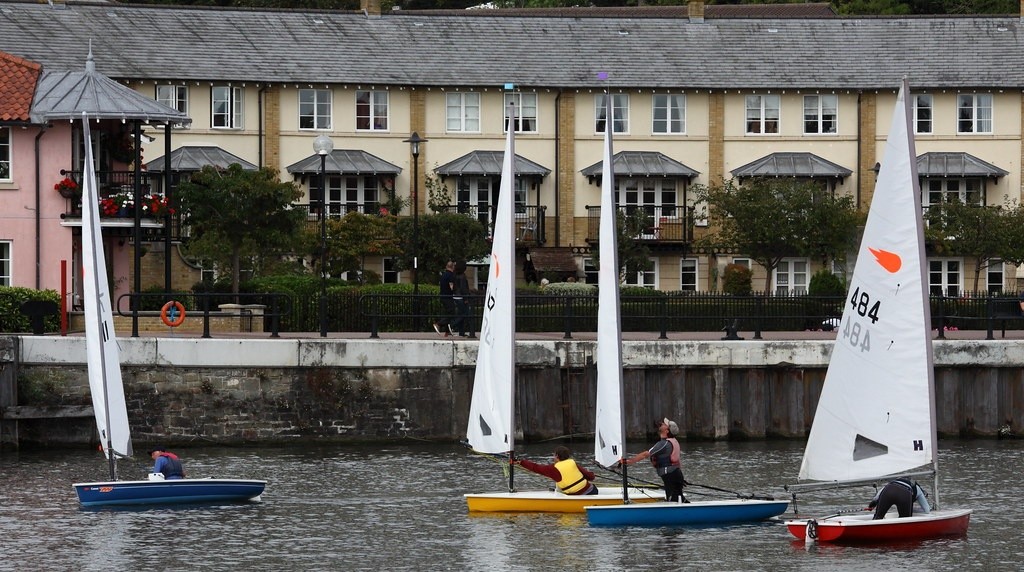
<box><xmin>513</xmin><ymin>446</ymin><xmax>598</xmax><ymax>495</ymax></box>
<box><xmin>147</xmin><ymin>446</ymin><xmax>188</xmax><ymax>480</ymax></box>
<box><xmin>865</xmin><ymin>476</ymin><xmax>930</xmax><ymax>519</ymax></box>
<box><xmin>619</xmin><ymin>416</ymin><xmax>691</xmax><ymax>504</ymax></box>
<box><xmin>433</xmin><ymin>257</ymin><xmax>470</xmax><ymax>338</ymax></box>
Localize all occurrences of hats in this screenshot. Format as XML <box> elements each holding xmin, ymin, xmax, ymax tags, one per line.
<box><xmin>147</xmin><ymin>446</ymin><xmax>165</xmax><ymax>456</ymax></box>
<box><xmin>664</xmin><ymin>418</ymin><xmax>679</xmax><ymax>435</ymax></box>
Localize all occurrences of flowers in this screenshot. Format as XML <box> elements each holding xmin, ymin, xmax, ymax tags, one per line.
<box><xmin>55</xmin><ymin>178</ymin><xmax>76</xmax><ymax>191</ymax></box>
<box><xmin>76</xmin><ymin>190</ymin><xmax>176</xmax><ymax>219</ymax></box>
<box><xmin>111</xmin><ymin>133</ymin><xmax>148</xmax><ymax>171</ymax></box>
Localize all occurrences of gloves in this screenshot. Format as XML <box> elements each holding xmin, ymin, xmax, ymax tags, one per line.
<box><xmin>868</xmin><ymin>500</ymin><xmax>877</xmax><ymax>512</ymax></box>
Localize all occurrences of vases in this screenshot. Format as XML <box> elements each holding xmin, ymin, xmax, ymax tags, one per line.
<box><xmin>59</xmin><ymin>189</ymin><xmax>75</xmax><ymax>197</ymax></box>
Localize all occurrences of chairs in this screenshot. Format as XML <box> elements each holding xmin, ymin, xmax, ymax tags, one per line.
<box><xmin>649</xmin><ymin>217</ymin><xmax>668</xmax><ymax>239</ymax></box>
<box><xmin>520</xmin><ymin>220</ymin><xmax>537</xmax><ymax>241</ymax></box>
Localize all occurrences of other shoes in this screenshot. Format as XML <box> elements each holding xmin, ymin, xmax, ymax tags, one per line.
<box><xmin>459</xmin><ymin>333</ymin><xmax>468</xmax><ymax>336</ymax></box>
<box><xmin>467</xmin><ymin>334</ymin><xmax>477</xmax><ymax>338</ymax></box>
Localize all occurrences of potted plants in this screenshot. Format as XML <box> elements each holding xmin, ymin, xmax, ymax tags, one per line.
<box><xmin>140</xmin><ymin>246</ymin><xmax>148</xmax><ymax>258</ymax></box>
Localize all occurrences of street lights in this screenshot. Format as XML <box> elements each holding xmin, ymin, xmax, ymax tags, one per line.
<box><xmin>402</xmin><ymin>130</ymin><xmax>429</xmax><ymax>330</ymax></box>
<box><xmin>312</xmin><ymin>132</ymin><xmax>334</xmax><ymax>338</ymax></box>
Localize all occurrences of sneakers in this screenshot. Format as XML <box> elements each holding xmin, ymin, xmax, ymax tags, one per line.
<box><xmin>433</xmin><ymin>324</ymin><xmax>441</xmax><ymax>335</ymax></box>
<box><xmin>448</xmin><ymin>324</ymin><xmax>454</xmax><ymax>336</ymax></box>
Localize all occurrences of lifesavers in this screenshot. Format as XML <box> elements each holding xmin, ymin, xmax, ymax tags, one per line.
<box><xmin>161</xmin><ymin>301</ymin><xmax>186</xmax><ymax>327</ymax></box>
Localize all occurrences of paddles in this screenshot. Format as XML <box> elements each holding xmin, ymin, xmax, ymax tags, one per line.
<box><xmin>836</xmin><ymin>507</ymin><xmax>874</xmax><ymax>514</ymax></box>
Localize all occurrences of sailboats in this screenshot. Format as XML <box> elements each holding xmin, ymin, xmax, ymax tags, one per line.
<box><xmin>581</xmin><ymin>86</ymin><xmax>790</xmax><ymax>527</ymax></box>
<box><xmin>461</xmin><ymin>106</ymin><xmax>680</xmax><ymax>515</ymax></box>
<box><xmin>783</xmin><ymin>74</ymin><xmax>971</xmax><ymax>544</ymax></box>
<box><xmin>71</xmin><ymin>111</ymin><xmax>268</xmax><ymax>508</ymax></box>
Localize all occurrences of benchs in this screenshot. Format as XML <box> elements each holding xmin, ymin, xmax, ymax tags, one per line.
<box><xmin>988</xmin><ymin>298</ymin><xmax>1024</xmax><ymax>338</ymax></box>
